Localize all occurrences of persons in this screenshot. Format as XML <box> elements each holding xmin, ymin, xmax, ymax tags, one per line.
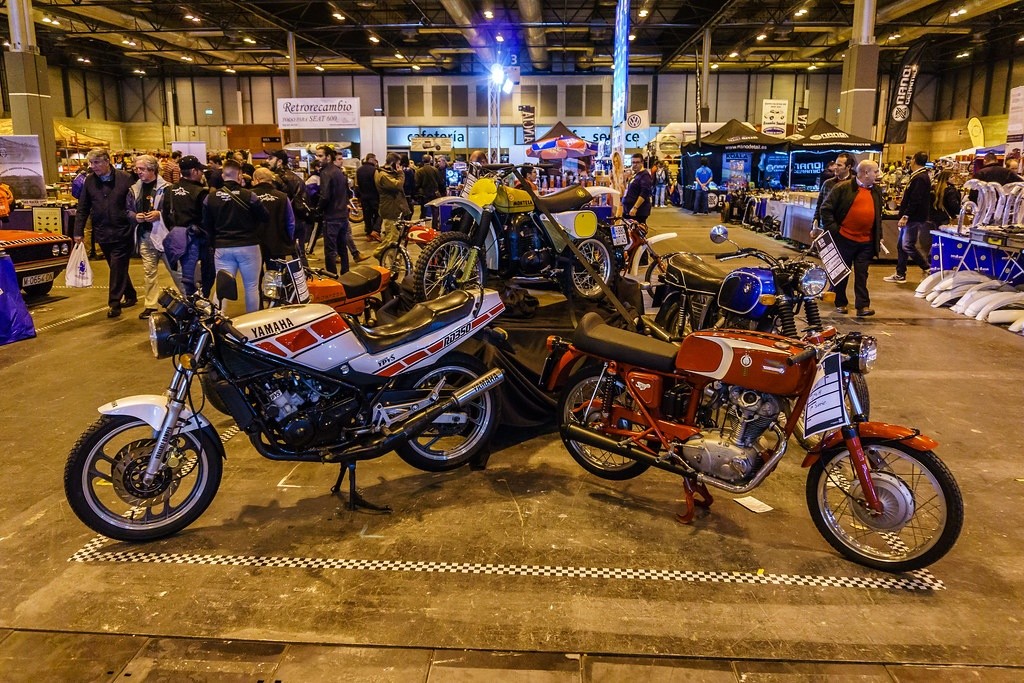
<box><xmin>968</xmin><ymin>152</ymin><xmax>1024</xmax><ymax>211</ymax></box>
<box><xmin>622</xmin><ymin>153</ymin><xmax>674</xmax><ymax>267</ymax></box>
<box><xmin>123</xmin><ymin>145</ymin><xmax>371</xmax><ymax>319</ymax></box>
<box><xmin>780</xmin><ymin>165</ymin><xmax>794</xmax><ymax>188</ymax></box>
<box><xmin>76</xmin><ymin>162</ymin><xmax>104</xmax><ymax>261</ymax></box>
<box><xmin>610</xmin><ymin>152</ymin><xmax>624</xmax><ymax>192</ymax></box>
<box><xmin>819</xmin><ymin>160</ymin><xmax>884</xmax><ymax>316</ymax></box>
<box><xmin>882</xmin><ymin>150</ymin><xmax>962</xmax><ymax>283</ymax></box>
<box><xmin>809</xmin><ymin>152</ymin><xmax>856</xmax><ymax>292</ymax></box>
<box><xmin>354</xmin><ymin>150</ymin><xmax>488</xmax><ymax>252</ymax></box>
<box><xmin>73</xmin><ymin>148</ymin><xmax>138</xmax><ymax>318</ymax></box>
<box><xmin>692</xmin><ymin>157</ymin><xmax>713</xmax><ymax>215</ymax></box>
<box><xmin>516</xmin><ymin>165</ymin><xmax>538</xmax><ymax>190</ymax></box>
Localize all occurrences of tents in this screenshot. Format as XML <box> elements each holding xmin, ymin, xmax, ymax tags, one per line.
<box><xmin>528</xmin><ymin>121</ymin><xmax>573</xmax><ymax>145</ymax></box>
<box><xmin>975</xmin><ymin>143</ymin><xmax>1005</xmax><ymax>156</ymax></box>
<box><xmin>648</xmin><ymin>118</ymin><xmax>883</xmax><ymax>209</ymax></box>
<box><xmin>0</xmin><ymin>118</ymin><xmax>110</xmax><ymax>182</ymax></box>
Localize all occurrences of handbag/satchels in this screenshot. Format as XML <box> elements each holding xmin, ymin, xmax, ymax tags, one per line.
<box><xmin>65</xmin><ymin>241</ymin><xmax>93</xmax><ymax>289</ymax></box>
<box><xmin>150</xmin><ymin>211</ymin><xmax>169</xmax><ymax>252</ymax></box>
<box><xmin>0</xmin><ymin>255</ymin><xmax>37</xmax><ymax>346</ymax></box>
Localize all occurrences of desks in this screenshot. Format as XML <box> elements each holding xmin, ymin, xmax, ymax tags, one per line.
<box><xmin>757</xmin><ymin>198</ymin><xmax>937</xmax><ymax>264</ymax></box>
<box><xmin>930</xmin><ymin>229</ymin><xmax>1024</xmax><ymax>282</ymax></box>
<box><xmin>683</xmin><ymin>187</ymin><xmax>728</xmax><ymax>214</ymax></box>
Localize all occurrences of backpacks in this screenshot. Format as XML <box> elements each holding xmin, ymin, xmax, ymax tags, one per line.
<box><xmin>656</xmin><ymin>170</ymin><xmax>665</xmax><ymax>183</ymax></box>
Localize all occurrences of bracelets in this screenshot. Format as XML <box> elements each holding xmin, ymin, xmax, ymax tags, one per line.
<box><xmin>634</xmin><ymin>206</ymin><xmax>638</xmax><ymax>209</ymax></box>
<box><xmin>903</xmin><ymin>216</ymin><xmax>907</xmax><ymax>218</ymax></box>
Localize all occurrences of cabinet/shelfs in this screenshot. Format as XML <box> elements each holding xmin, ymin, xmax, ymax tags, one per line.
<box><xmin>56</xmin><ymin>145</ymin><xmax>111</xmax><ymax>185</ymax></box>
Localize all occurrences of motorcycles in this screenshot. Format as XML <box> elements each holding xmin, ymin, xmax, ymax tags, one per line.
<box><xmin>639</xmin><ymin>226</ymin><xmax>870</xmax><ymax>452</ymax></box>
<box><xmin>64</xmin><ymin>268</ymin><xmax>516</xmax><ymax>543</ymax></box>
<box><xmin>538</xmin><ymin>313</ymin><xmax>965</xmax><ymax>575</ymax></box>
<box><xmin>258</xmin><ymin>162</ymin><xmax>672</xmax><ymax>323</ymax></box>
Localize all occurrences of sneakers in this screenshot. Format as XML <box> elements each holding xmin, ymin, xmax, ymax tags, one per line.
<box><xmin>882</xmin><ymin>271</ymin><xmax>907</xmax><ymax>284</ymax></box>
<box><xmin>918</xmin><ymin>269</ymin><xmax>931</xmax><ymax>285</ymax></box>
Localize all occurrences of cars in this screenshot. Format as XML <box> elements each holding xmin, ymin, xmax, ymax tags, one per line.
<box><xmin>0</xmin><ymin>230</ymin><xmax>74</xmax><ymax>302</ymax></box>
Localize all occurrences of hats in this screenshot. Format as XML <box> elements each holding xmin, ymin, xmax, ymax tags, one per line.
<box><xmin>179</xmin><ymin>155</ymin><xmax>209</xmax><ymax>170</ymax></box>
<box><xmin>263</xmin><ymin>148</ymin><xmax>289</xmax><ymax>164</ymax></box>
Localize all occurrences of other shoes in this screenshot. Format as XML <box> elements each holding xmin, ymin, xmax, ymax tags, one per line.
<box><xmin>660</xmin><ymin>205</ymin><xmax>668</xmax><ymax>208</ymax></box>
<box><xmin>654</xmin><ymin>204</ymin><xmax>659</xmax><ymax>208</ymax></box>
<box><xmin>692</xmin><ymin>211</ymin><xmax>697</xmax><ymax>215</ymax></box>
<box><xmin>370</xmin><ymin>230</ymin><xmax>383</xmax><ymax>243</ymax></box>
<box><xmin>372</xmin><ymin>252</ymin><xmax>386</xmax><ymax>267</ymax></box>
<box><xmin>703</xmin><ymin>211</ymin><xmax>708</xmax><ymax>215</ymax></box>
<box><xmin>353</xmin><ymin>254</ymin><xmax>371</xmax><ymax>263</ymax></box>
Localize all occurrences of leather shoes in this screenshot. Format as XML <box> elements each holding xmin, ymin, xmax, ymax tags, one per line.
<box><xmin>139</xmin><ymin>308</ymin><xmax>158</xmax><ymax>319</ymax></box>
<box><xmin>836</xmin><ymin>305</ymin><xmax>848</xmax><ymax>314</ymax></box>
<box><xmin>121</xmin><ymin>296</ymin><xmax>138</xmax><ymax>307</ymax></box>
<box><xmin>855</xmin><ymin>306</ymin><xmax>875</xmax><ymax>316</ymax></box>
<box><xmin>108</xmin><ymin>305</ymin><xmax>121</xmax><ymax>317</ymax></box>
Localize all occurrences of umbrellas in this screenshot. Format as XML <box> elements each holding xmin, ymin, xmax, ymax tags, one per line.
<box><xmin>525</xmin><ymin>133</ymin><xmax>599</xmax><ymax>175</ymax></box>
